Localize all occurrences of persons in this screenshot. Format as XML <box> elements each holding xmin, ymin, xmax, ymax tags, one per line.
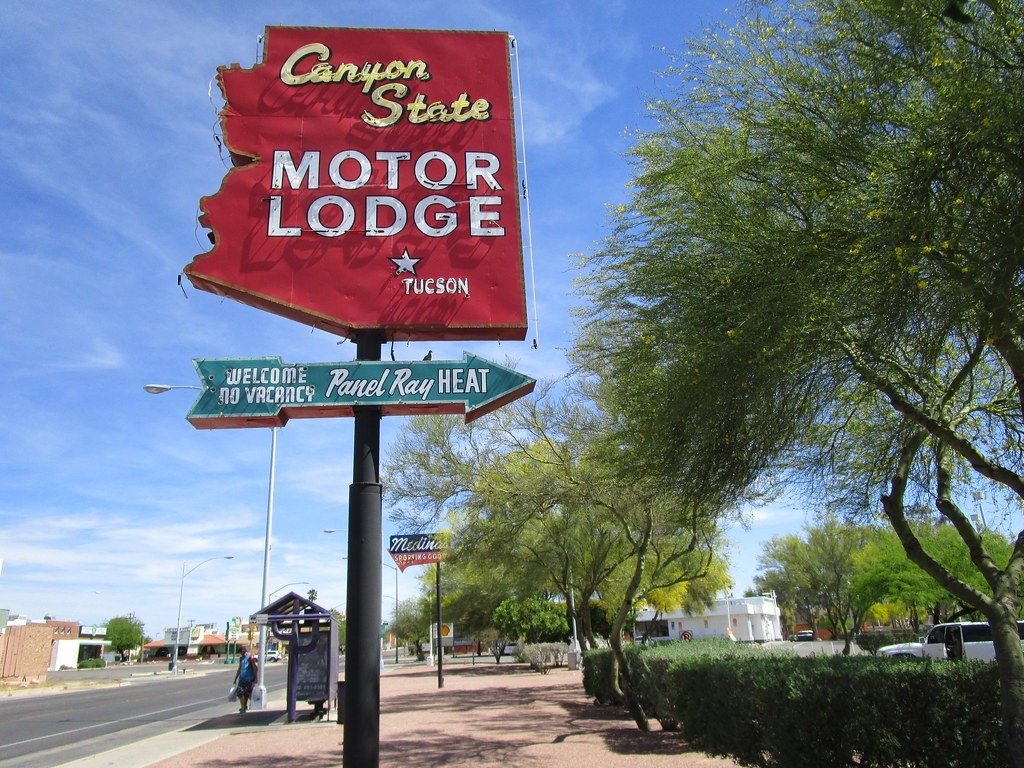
<box><xmin>946</xmin><ymin>629</ymin><xmax>962</xmax><ymax>658</ymax></box>
<box><xmin>233</xmin><ymin>646</ymin><xmax>257</xmax><ymax>714</ymax></box>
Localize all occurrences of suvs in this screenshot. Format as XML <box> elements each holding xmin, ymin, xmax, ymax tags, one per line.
<box><xmin>503</xmin><ymin>642</ymin><xmax>518</xmax><ymax>655</ymax></box>
<box><xmin>253</xmin><ymin>651</ymin><xmax>282</xmax><ymax>663</ymax></box>
<box><xmin>876</xmin><ymin>618</ymin><xmax>1024</xmax><ymax>663</ymax></box>
<box><xmin>789</xmin><ymin>630</ymin><xmax>814</xmax><ymax>637</ymax></box>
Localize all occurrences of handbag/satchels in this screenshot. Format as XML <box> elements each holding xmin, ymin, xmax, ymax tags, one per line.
<box><xmin>227</xmin><ymin>683</ymin><xmax>237</xmax><ymax>702</ymax></box>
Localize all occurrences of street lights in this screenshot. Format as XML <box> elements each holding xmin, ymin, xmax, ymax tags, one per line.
<box><xmin>171</xmin><ymin>555</ymin><xmax>233</xmax><ymax>674</ymax></box>
<box><xmin>384</xmin><ymin>595</ymin><xmax>405</xmax><ymax>657</ymax></box>
<box><xmin>187</xmin><ymin>620</ymin><xmax>195</xmax><ymax>626</ymax></box>
<box><xmin>143</xmin><ymin>383</ymin><xmax>277</xmax><ymax>708</ymax></box>
<box><xmin>268</xmin><ymin>581</ymin><xmax>310</xmax><ymax>605</ymax></box>
<box><xmin>342</xmin><ymin>556</ymin><xmax>399</xmax><ymax>665</ymax></box>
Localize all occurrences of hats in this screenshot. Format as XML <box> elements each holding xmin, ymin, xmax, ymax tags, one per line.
<box><xmin>236</xmin><ymin>646</ymin><xmax>248</xmax><ymax>653</ymax></box>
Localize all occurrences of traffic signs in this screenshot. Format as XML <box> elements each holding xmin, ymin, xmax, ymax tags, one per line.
<box><xmin>183</xmin><ymin>351</ymin><xmax>537</xmax><ymax>431</ymax></box>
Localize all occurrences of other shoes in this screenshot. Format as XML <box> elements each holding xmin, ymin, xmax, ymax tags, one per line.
<box><xmin>239</xmin><ymin>706</ymin><xmax>247</xmax><ymax>711</ymax></box>
<box><xmin>240</xmin><ymin>708</ymin><xmax>245</xmax><ymax>714</ymax></box>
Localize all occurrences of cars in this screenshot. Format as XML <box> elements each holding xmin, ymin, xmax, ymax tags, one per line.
<box><xmin>635</xmin><ymin>636</ymin><xmax>643</xmax><ymax>646</ymax></box>
